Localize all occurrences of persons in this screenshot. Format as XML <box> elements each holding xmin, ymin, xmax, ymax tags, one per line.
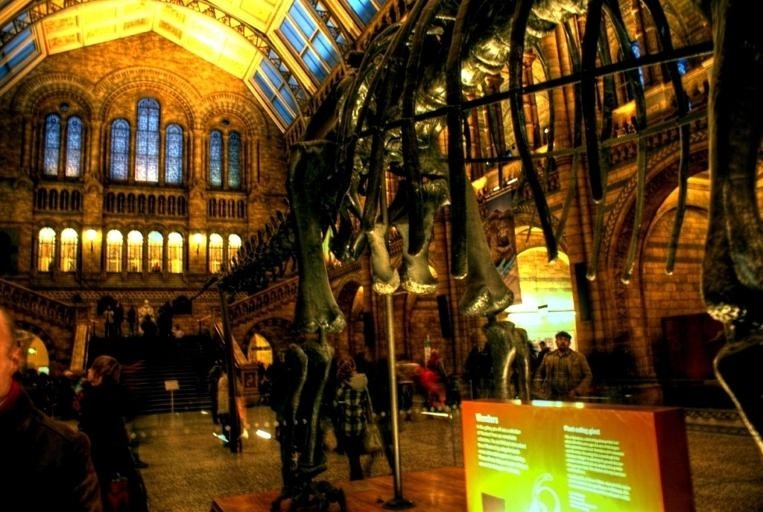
<box><xmin>206</xmin><ymin>357</ymin><xmax>242</xmax><ymax>455</ymax></box>
<box><xmin>0</xmin><ymin>305</ymin><xmax>148</xmax><ymax>512</ymax></box>
<box><xmin>140</xmin><ymin>313</ymin><xmax>158</xmax><ymax>348</ymax></box>
<box><xmin>138</xmin><ymin>298</ymin><xmax>154</xmax><ymax>323</ymax></box>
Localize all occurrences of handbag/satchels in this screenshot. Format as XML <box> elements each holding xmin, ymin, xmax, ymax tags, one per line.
<box><xmin>360</xmin><ymin>423</ymin><xmax>383</xmax><ymax>455</ymax></box>
